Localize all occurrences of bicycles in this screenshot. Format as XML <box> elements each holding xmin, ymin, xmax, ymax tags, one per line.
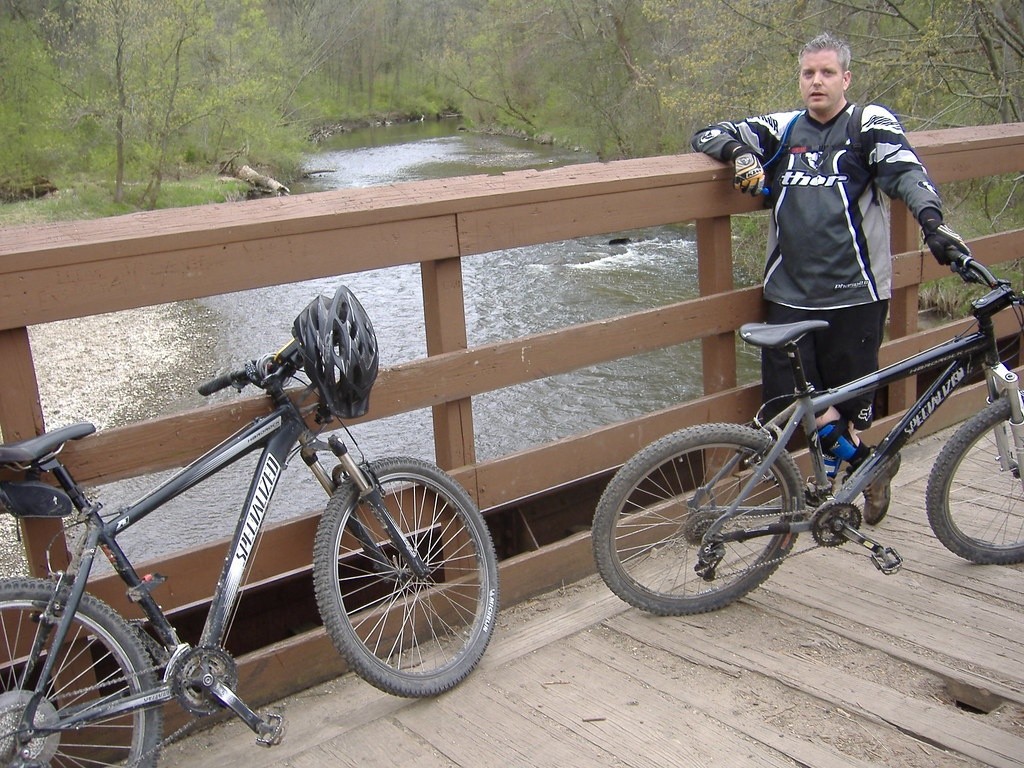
<box><xmin>590</xmin><ymin>246</ymin><xmax>1024</xmax><ymax>617</ymax></box>
<box><xmin>1</xmin><ymin>319</ymin><xmax>503</xmax><ymax>768</ymax></box>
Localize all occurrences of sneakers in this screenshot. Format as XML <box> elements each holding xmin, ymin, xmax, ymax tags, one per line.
<box><xmin>843</xmin><ymin>447</ymin><xmax>902</xmax><ymax>526</ymax></box>
<box><xmin>804</xmin><ymin>476</ymin><xmax>835</xmax><ymax>507</ymax></box>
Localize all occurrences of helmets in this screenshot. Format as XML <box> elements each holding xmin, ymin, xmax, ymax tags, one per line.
<box><xmin>291</xmin><ymin>285</ymin><xmax>379</xmax><ymax>418</ymax></box>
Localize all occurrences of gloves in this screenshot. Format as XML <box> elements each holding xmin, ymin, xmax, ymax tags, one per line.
<box><xmin>731</xmin><ymin>146</ymin><xmax>766</xmax><ymax>197</ymax></box>
<box><xmin>920</xmin><ymin>218</ymin><xmax>973</xmax><ymax>274</ymax></box>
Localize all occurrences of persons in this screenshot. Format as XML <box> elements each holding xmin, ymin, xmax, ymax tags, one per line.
<box><xmin>691</xmin><ymin>35</ymin><xmax>972</xmax><ymax>526</ymax></box>
